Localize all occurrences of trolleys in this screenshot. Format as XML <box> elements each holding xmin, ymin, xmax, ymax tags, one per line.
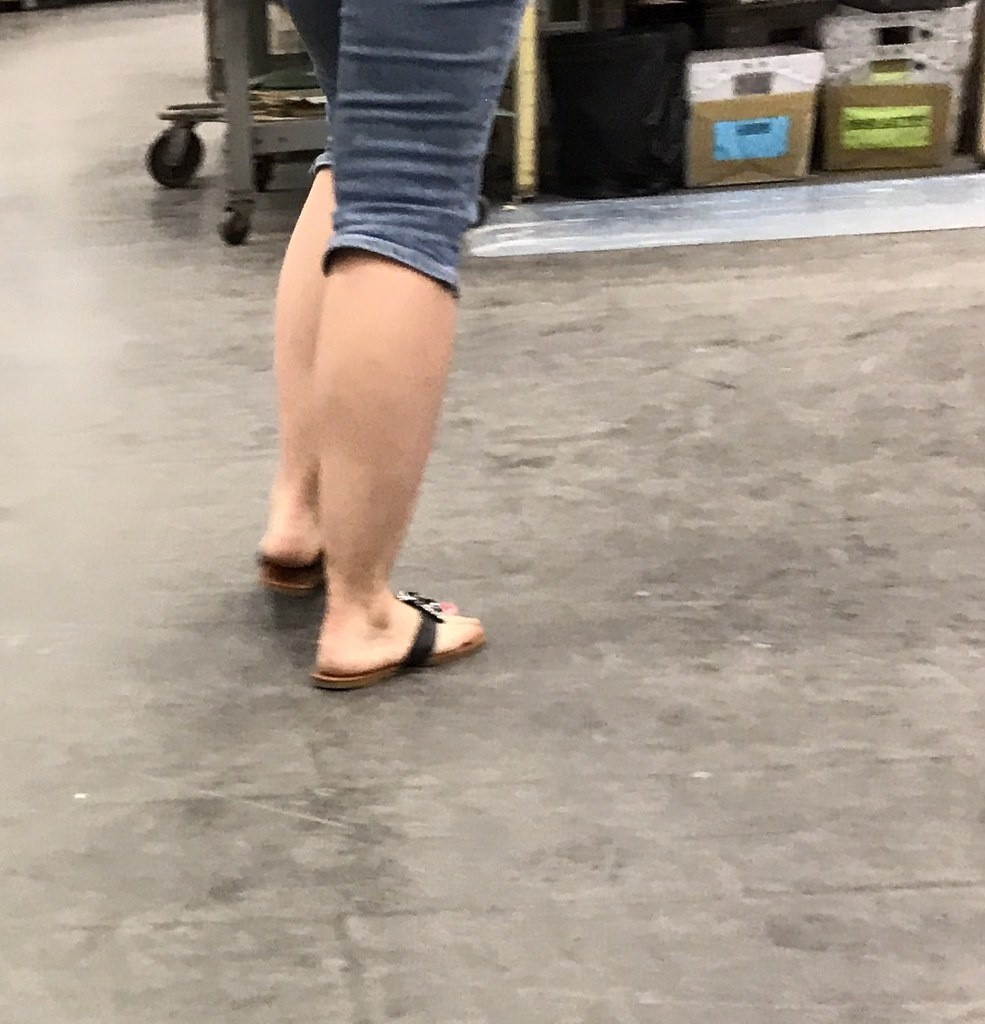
<box><xmin>145</xmin><ymin>1</ymin><xmax>491</xmax><ymax>246</ymax></box>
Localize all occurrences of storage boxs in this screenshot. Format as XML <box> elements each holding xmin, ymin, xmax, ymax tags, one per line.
<box><xmin>684</xmin><ymin>47</ymin><xmax>824</xmax><ymax>190</ymax></box>
<box><xmin>820</xmin><ymin>0</ymin><xmax>978</xmax><ymax>171</ymax></box>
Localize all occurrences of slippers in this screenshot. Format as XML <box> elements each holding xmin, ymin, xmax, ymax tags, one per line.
<box><xmin>309</xmin><ymin>589</ymin><xmax>485</xmax><ymax>690</ymax></box>
<box><xmin>263</xmin><ymin>549</ymin><xmax>326</xmax><ymax>596</ymax></box>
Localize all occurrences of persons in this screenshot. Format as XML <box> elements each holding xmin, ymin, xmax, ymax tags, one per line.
<box><xmin>264</xmin><ymin>0</ymin><xmax>525</xmax><ymax>692</ymax></box>
